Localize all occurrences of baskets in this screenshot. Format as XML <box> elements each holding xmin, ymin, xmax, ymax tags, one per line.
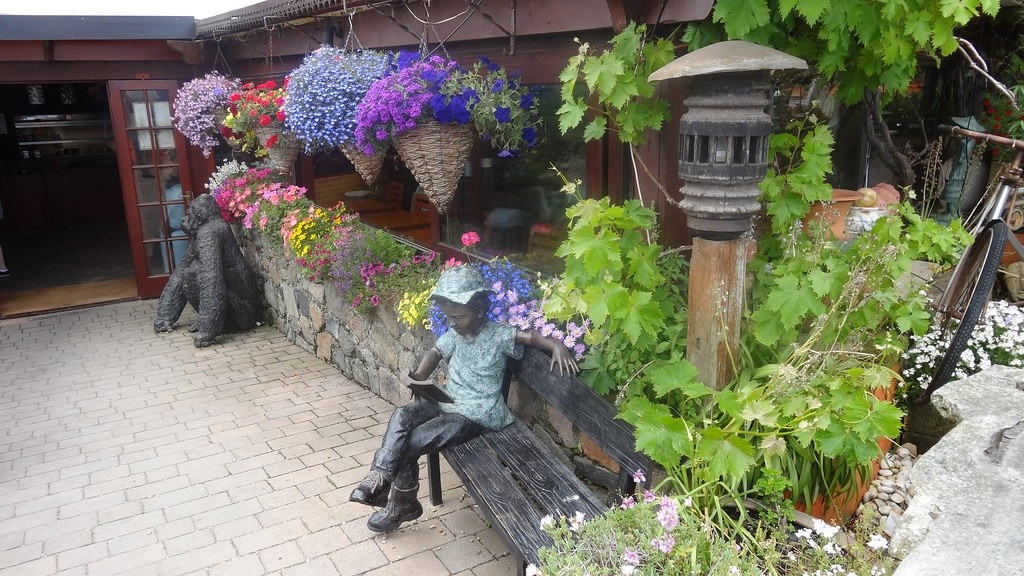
<box><xmin>257</xmin><ymin>128</ymin><xmax>299</xmax><ymax>177</ymax></box>
<box><xmin>390</xmin><ymin>115</ymin><xmax>475</xmax><ymax>215</ymax></box>
<box><xmin>214</xmin><ymin>106</ymin><xmax>246</xmax><ymax>151</ymax></box>
<box><xmin>338</xmin><ymin>140</ymin><xmax>389</xmax><ymax>184</ymax></box>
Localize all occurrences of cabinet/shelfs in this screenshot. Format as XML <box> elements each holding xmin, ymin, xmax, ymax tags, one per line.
<box><xmin>13</xmin><ymin>119</ymin><xmax>117</xmax><ymax>167</ymax></box>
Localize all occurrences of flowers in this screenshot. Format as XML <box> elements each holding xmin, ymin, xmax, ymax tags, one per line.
<box><xmin>352</xmin><ymin>52</ymin><xmax>545</xmax><ymax>156</ymax></box>
<box><xmin>524</xmin><ymin>467</ymin><xmax>896</xmax><ymax>575</ymax></box>
<box><xmin>170</xmin><ymin>71</ymin><xmax>242</xmax><ymax>159</ymax></box>
<box><xmin>282</xmin><ymin>43</ymin><xmax>391</xmax><ymax>157</ymax></box>
<box><xmin>982</xmin><ymin>93</ymin><xmax>1024</xmax><ymax>154</ymax></box>
<box><xmin>218</xmin><ymin>76</ymin><xmax>293</xmax><ymax>147</ymax></box>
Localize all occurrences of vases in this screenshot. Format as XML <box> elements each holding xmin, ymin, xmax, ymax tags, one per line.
<box><xmin>391</xmin><ymin>116</ymin><xmax>476</xmax><ymax>214</ymax></box>
<box><xmin>803</xmin><ymin>190</ymin><xmax>865</xmax><ymax>241</ymax></box>
<box><xmin>256</xmin><ymin>122</ymin><xmax>299</xmax><ymax>174</ymax></box>
<box><xmin>340</xmin><ymin>142</ymin><xmax>389</xmax><ymax>185</ymax></box>
<box><xmin>713</xmin><ymin>499</ymin><xmax>888</xmax><ymax>575</ymax></box>
<box><xmin>215</xmin><ymin>112</ymin><xmax>242</xmax><ymax>150</ymax></box>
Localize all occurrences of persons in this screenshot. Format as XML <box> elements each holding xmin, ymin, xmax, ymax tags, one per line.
<box><xmin>0</xmin><ymin>200</ymin><xmax>11</xmax><ymax>278</ymax></box>
<box><xmin>348</xmin><ymin>264</ymin><xmax>580</xmax><ymax>534</ymax></box>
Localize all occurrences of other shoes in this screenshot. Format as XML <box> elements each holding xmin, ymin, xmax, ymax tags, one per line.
<box><xmin>0</xmin><ymin>269</ymin><xmax>10</xmax><ymax>277</ymax></box>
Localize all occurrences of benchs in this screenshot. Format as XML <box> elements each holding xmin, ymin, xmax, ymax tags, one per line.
<box><xmin>426</xmin><ymin>345</ymin><xmax>654</xmax><ymax>575</ymax></box>
